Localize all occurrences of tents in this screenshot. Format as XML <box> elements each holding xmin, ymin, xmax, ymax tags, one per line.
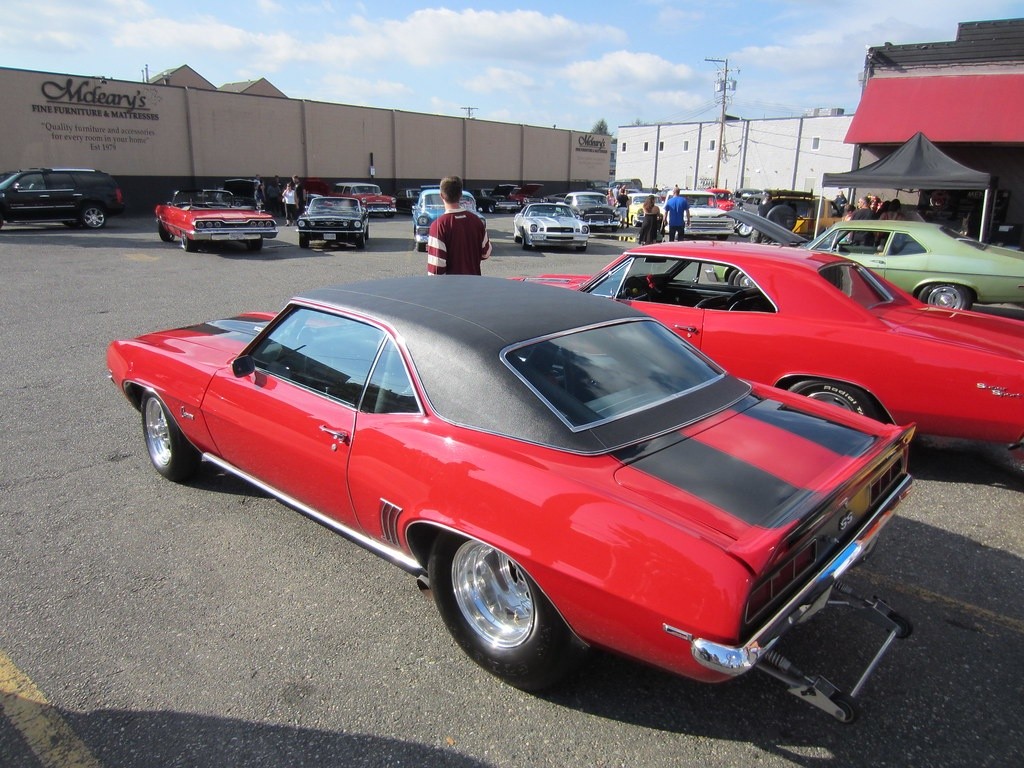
<box><xmin>814</xmin><ymin>132</ymin><xmax>1000</xmax><ymax>240</ymax></box>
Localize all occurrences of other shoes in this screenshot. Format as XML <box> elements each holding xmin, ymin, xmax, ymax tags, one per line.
<box><xmin>286</xmin><ymin>223</ymin><xmax>290</xmax><ymax>226</ymax></box>
<box><xmin>620</xmin><ymin>226</ymin><xmax>624</xmax><ymax>229</ymax></box>
<box><xmin>626</xmin><ymin>226</ymin><xmax>629</xmax><ymax>228</ymax></box>
<box><xmin>292</xmin><ymin>222</ymin><xmax>295</xmax><ymax>226</ymax></box>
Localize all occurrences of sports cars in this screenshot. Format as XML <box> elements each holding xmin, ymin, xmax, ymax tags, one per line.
<box><xmin>498</xmin><ymin>241</ymin><xmax>1024</xmax><ymax>443</ymax></box>
<box><xmin>108</xmin><ymin>274</ymin><xmax>918</xmax><ymax>722</ymax></box>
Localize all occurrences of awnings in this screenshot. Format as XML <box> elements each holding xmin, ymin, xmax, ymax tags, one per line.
<box><xmin>843</xmin><ymin>76</ymin><xmax>1024</xmax><ymax>145</ymax></box>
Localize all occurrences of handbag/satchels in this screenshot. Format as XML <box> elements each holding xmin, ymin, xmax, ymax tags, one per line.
<box><xmin>282</xmin><ymin>197</ymin><xmax>286</xmax><ymax>203</ymax></box>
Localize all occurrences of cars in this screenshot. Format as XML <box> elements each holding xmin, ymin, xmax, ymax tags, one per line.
<box><xmin>478</xmin><ymin>182</ymin><xmax>852</xmax><ymax>252</ymax></box>
<box><xmin>724</xmin><ymin>209</ymin><xmax>1024</xmax><ymax>310</ymax></box>
<box><xmin>155</xmin><ymin>178</ymin><xmax>279</xmax><ymax>254</ymax></box>
<box><xmin>0</xmin><ymin>168</ymin><xmax>125</xmax><ymax>228</ymax></box>
<box><xmin>391</xmin><ymin>185</ymin><xmax>485</xmax><ymax>254</ymax></box>
<box><xmin>296</xmin><ymin>196</ymin><xmax>370</xmax><ymax>248</ymax></box>
<box><xmin>334</xmin><ymin>182</ymin><xmax>398</xmax><ymax>218</ymax></box>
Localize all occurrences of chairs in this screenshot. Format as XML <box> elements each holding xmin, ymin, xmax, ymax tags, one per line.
<box><xmin>727</xmin><ymin>288</ymin><xmax>768</xmax><ymax>307</ymax></box>
<box><xmin>695</xmin><ymin>295</ymin><xmax>727</xmax><ymax>310</ymax></box>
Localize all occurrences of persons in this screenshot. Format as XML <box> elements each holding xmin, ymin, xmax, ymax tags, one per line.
<box><xmin>662</xmin><ymin>188</ymin><xmax>691</xmax><ymax>242</ymax></box>
<box><xmin>879</xmin><ymin>199</ymin><xmax>906</xmax><ymax>221</ymax></box>
<box><xmin>606</xmin><ymin>184</ymin><xmax>630</xmax><ymax>229</ymax></box>
<box><xmin>850</xmin><ymin>196</ymin><xmax>879</xmax><ymax>220</ymax></box>
<box><xmin>254</xmin><ymin>174</ymin><xmax>306</xmax><ymax>226</ymax></box>
<box><xmin>842</xmin><ymin>205</ymin><xmax>856</xmax><ymax>221</ymax></box>
<box><xmin>429</xmin><ymin>175</ymin><xmax>492</xmax><ymax>275</ymax></box>
<box><xmin>638</xmin><ymin>195</ymin><xmax>663</xmax><ymax>246</ymax></box>
<box><xmin>835</xmin><ymin>191</ymin><xmax>847</xmax><ymax>213</ymax></box>
<box><xmin>857</xmin><ymin>192</ymin><xmax>890</xmax><ymax>217</ymax></box>
